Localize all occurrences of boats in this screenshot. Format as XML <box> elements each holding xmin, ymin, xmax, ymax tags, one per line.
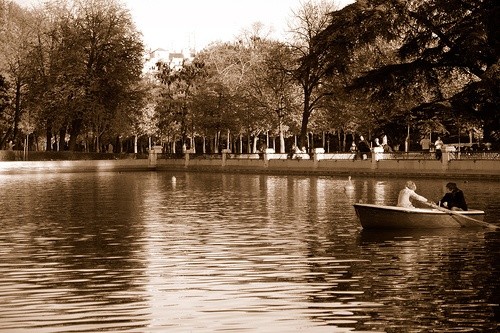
<box><xmin>352</xmin><ymin>201</ymin><xmax>485</xmax><ymax>230</ymax></box>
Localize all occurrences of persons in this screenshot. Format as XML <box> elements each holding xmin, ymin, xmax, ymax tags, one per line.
<box><xmin>420</xmin><ymin>135</ymin><xmax>431</xmax><ymax>156</ymax></box>
<box><xmin>434</xmin><ymin>136</ymin><xmax>444</xmax><ymax>163</ymax></box>
<box><xmin>357</xmin><ymin>134</ymin><xmax>393</xmax><ymax>160</ymax></box>
<box><xmin>434</xmin><ymin>183</ymin><xmax>468</xmax><ymax>212</ymax></box>
<box><xmin>53</xmin><ymin>141</ymin><xmax>57</xmax><ymax>151</ymax></box>
<box><xmin>398</xmin><ymin>180</ymin><xmax>432</xmax><ymax>209</ymax></box>
<box><xmin>106</xmin><ymin>141</ymin><xmax>113</xmax><ymax>153</ymax></box>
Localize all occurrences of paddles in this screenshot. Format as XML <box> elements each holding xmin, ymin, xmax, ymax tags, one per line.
<box><xmin>422</xmin><ymin>201</ymin><xmax>500</xmax><ymax>232</ymax></box>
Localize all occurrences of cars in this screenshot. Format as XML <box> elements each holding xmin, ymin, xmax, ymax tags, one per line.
<box><xmin>443</xmin><ymin>142</ymin><xmax>495</xmax><ymax>153</ymax></box>
